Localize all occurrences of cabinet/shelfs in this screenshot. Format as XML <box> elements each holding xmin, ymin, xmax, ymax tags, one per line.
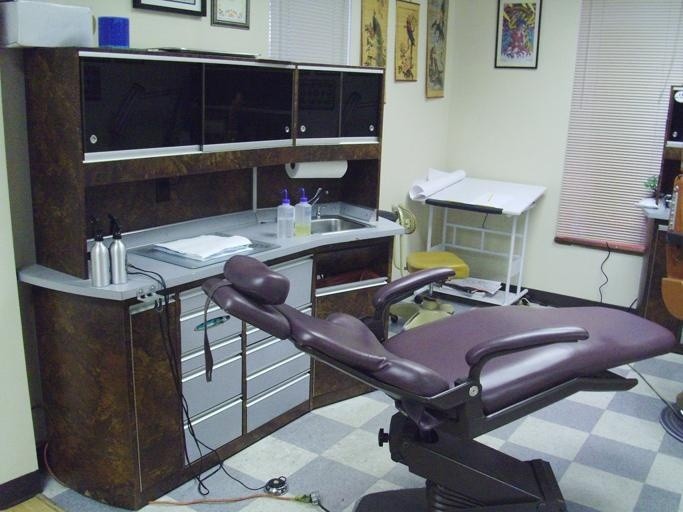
<box><xmin>178</xmin><ymin>254</ymin><xmax>315</xmax><ymax>483</ymax></box>
<box><xmin>23</xmin><ymin>43</ymin><xmax>387</xmax><ymax>185</ymax></box>
<box><xmin>310</xmin><ymin>235</ymin><xmax>394</xmax><ymax>410</ymax></box>
<box><xmin>635</xmin><ymin>85</ymin><xmax>682</xmax><ymax>354</ymax></box>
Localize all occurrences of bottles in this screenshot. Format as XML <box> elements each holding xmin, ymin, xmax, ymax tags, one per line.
<box><xmin>276</xmin><ymin>188</ymin><xmax>311</xmax><ymax>239</ymax></box>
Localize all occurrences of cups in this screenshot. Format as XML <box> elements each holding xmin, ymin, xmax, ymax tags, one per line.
<box><xmin>96</xmin><ymin>15</ymin><xmax>129</xmax><ymax>49</ymax></box>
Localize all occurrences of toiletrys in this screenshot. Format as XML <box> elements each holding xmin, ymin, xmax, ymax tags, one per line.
<box><xmin>276</xmin><ymin>189</ymin><xmax>295</xmax><ymax>241</ymax></box>
<box><xmin>295</xmin><ymin>188</ymin><xmax>312</xmax><ymax>237</ymax></box>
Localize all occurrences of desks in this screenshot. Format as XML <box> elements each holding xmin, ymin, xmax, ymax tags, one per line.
<box><xmin>414</xmin><ymin>196</ymin><xmax>536</xmax><ymax>308</ymax></box>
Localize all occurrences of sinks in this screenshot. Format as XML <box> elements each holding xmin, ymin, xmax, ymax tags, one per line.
<box><xmin>311</xmin><ymin>215</ymin><xmax>375</xmax><ymax>234</ymax></box>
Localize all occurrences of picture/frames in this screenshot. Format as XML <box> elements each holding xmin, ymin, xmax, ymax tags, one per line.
<box><xmin>494</xmin><ymin>0</ymin><xmax>543</xmax><ymax>70</ymax></box>
<box><xmin>133</xmin><ymin>0</ymin><xmax>251</xmax><ymax>29</ymax></box>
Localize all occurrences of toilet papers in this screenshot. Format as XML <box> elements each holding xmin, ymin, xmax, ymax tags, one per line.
<box><xmin>285</xmin><ymin>160</ymin><xmax>348</xmax><ymax>178</ymax></box>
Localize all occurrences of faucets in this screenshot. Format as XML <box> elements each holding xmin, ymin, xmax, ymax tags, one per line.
<box><xmin>309</xmin><ymin>187</ymin><xmax>322</xmax><ymax>203</ymax></box>
<box><xmin>311</xmin><ymin>190</ymin><xmax>331</xmax><ymax>218</ymax></box>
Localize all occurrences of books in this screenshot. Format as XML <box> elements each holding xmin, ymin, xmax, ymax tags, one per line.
<box><xmin>443</xmin><ymin>284</ymin><xmax>476</xmax><ymax>294</ymax></box>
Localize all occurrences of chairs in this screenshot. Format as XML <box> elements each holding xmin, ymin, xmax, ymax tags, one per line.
<box><xmin>659</xmin><ymin>277</ymin><xmax>683</xmax><ymax>442</ymax></box>
<box><xmin>198</xmin><ymin>256</ymin><xmax>676</xmax><ymax>512</ymax></box>
